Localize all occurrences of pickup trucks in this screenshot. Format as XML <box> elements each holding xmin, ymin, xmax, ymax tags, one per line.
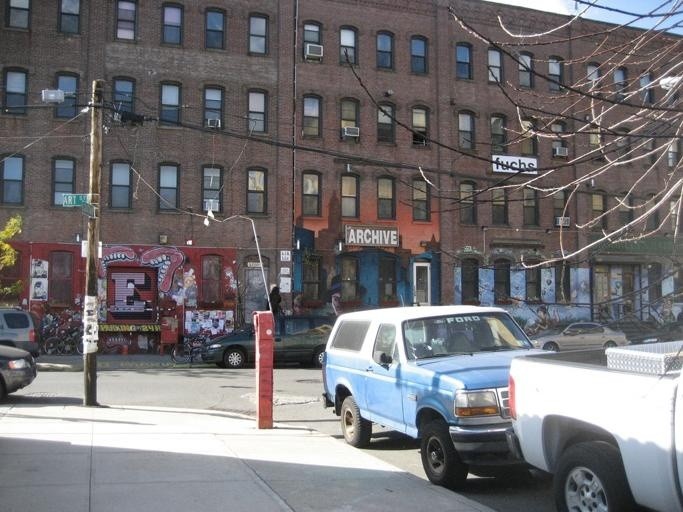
<box><xmin>509</xmin><ymin>338</ymin><xmax>682</xmax><ymax>512</ymax></box>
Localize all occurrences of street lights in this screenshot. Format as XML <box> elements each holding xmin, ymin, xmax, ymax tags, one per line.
<box><xmin>38</xmin><ymin>79</ymin><xmax>111</xmax><ymax>409</ymax></box>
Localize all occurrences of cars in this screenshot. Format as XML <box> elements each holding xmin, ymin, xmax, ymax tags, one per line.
<box><xmin>0</xmin><ymin>344</ymin><xmax>37</xmax><ymax>402</ymax></box>
<box><xmin>523</xmin><ymin>320</ymin><xmax>628</xmax><ymax>356</ymax></box>
<box><xmin>628</xmin><ymin>321</ymin><xmax>682</xmax><ymax>346</ymax></box>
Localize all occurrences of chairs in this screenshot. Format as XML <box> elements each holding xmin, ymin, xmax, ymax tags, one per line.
<box><xmin>447</xmin><ymin>332</ymin><xmax>474</xmax><ymax>352</ymax></box>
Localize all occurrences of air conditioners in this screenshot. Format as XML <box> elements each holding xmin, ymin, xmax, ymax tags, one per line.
<box><xmin>206</xmin><ymin>118</ymin><xmax>221</xmax><ymax>128</ymax></box>
<box><xmin>553</xmin><ymin>146</ymin><xmax>568</xmax><ymax>158</ymax></box>
<box><xmin>554</xmin><ymin>216</ymin><xmax>570</xmax><ymax>227</ymax></box>
<box><xmin>303</xmin><ymin>42</ymin><xmax>324</xmax><ymax>64</ymax></box>
<box><xmin>341</xmin><ymin>126</ymin><xmax>359</xmax><ymax>143</ymax></box>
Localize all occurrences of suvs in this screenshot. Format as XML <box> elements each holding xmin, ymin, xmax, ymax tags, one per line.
<box><xmin>200</xmin><ymin>310</ymin><xmax>339</xmax><ymax>369</ymax></box>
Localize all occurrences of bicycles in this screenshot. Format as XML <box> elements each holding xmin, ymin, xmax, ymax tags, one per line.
<box><xmin>169</xmin><ymin>333</ymin><xmax>207</xmax><ymax>363</ymax></box>
<box><xmin>42</xmin><ymin>313</ymin><xmax>101</xmax><ymax>356</ymax></box>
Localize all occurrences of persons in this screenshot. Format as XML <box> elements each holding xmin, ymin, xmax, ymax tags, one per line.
<box><xmin>265</xmin><ymin>287</ymin><xmax>282</xmax><ymax>314</ymax></box>
<box><xmin>534</xmin><ymin>305</ymin><xmax>561</xmax><ymax>334</ymax></box>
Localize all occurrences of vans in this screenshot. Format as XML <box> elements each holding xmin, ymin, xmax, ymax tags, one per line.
<box><xmin>0</xmin><ymin>307</ymin><xmax>40</xmax><ymax>359</ymax></box>
<box><xmin>319</xmin><ymin>305</ymin><xmax>534</xmax><ymax>486</ymax></box>
